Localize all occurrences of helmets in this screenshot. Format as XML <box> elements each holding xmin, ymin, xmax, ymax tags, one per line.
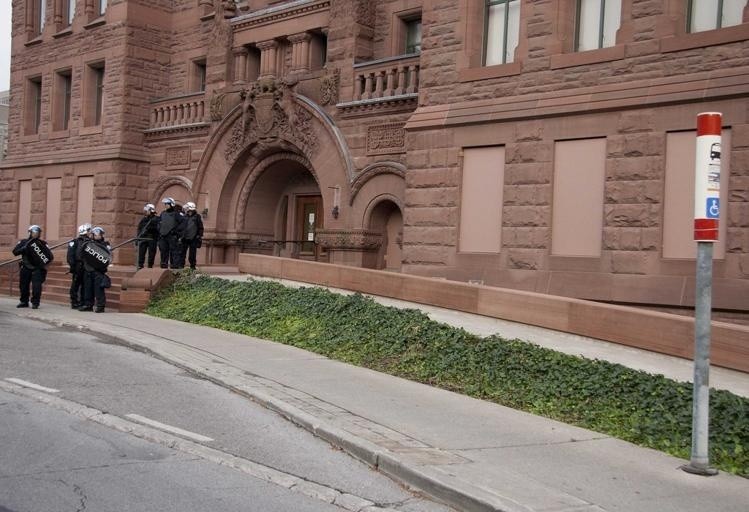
<box><xmin>79</xmin><ymin>225</ymin><xmax>104</xmax><ymax>235</ymax></box>
<box><xmin>184</xmin><ymin>202</ymin><xmax>196</xmax><ymax>212</ymax></box>
<box><xmin>28</xmin><ymin>225</ymin><xmax>41</xmax><ymax>233</ymax></box>
<box><xmin>163</xmin><ymin>198</ymin><xmax>175</xmax><ymax>207</ymax></box>
<box><xmin>144</xmin><ymin>204</ymin><xmax>155</xmax><ymax>212</ymax></box>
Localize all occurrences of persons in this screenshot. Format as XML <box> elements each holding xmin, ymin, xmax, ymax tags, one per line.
<box><xmin>66</xmin><ymin>224</ymin><xmax>94</xmax><ymax>310</ymax></box>
<box><xmin>79</xmin><ymin>226</ymin><xmax>111</xmax><ymax>313</ymax></box>
<box><xmin>134</xmin><ymin>204</ymin><xmax>161</xmax><ymax>269</ymax></box>
<box><xmin>158</xmin><ymin>197</ymin><xmax>185</xmax><ymax>268</ymax></box>
<box><xmin>11</xmin><ymin>224</ymin><xmax>52</xmax><ymax>309</ymax></box>
<box><xmin>173</xmin><ymin>205</ymin><xmax>187</xmax><ymax>269</ymax></box>
<box><xmin>178</xmin><ymin>201</ymin><xmax>205</xmax><ymax>271</ymax></box>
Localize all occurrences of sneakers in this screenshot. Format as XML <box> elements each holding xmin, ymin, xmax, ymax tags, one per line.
<box><xmin>72</xmin><ymin>303</ymin><xmax>104</xmax><ymax>313</ymax></box>
<box><xmin>17</xmin><ymin>303</ymin><xmax>37</xmax><ymax>308</ymax></box>
